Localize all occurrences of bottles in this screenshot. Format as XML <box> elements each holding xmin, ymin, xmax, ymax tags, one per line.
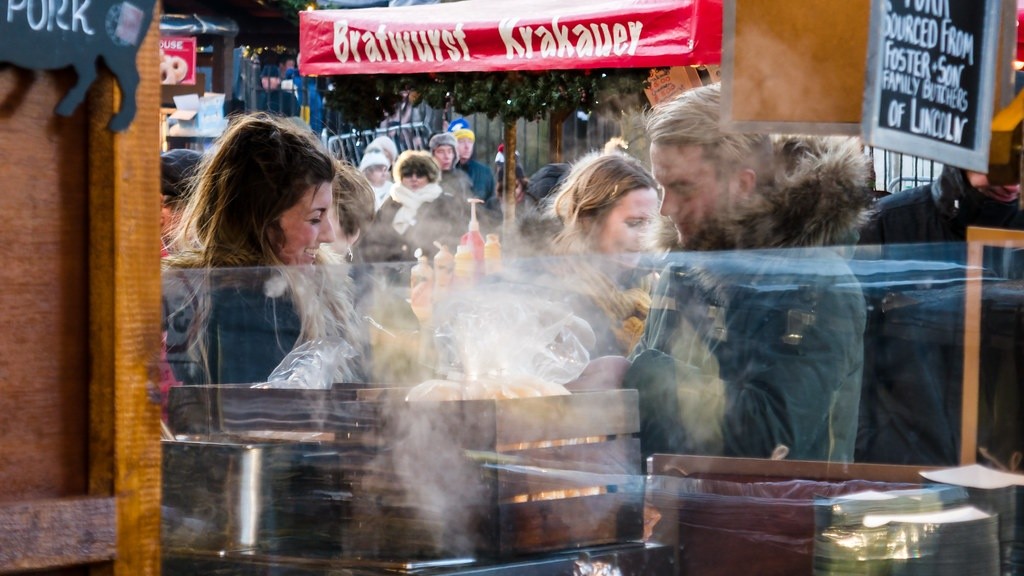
<box><xmin>410</xmin><ymin>199</ymin><xmax>501</xmax><ymax>323</ymax></box>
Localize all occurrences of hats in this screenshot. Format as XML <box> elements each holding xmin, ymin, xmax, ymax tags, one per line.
<box><xmin>393</xmin><ymin>150</ymin><xmax>441</xmax><ymax>187</ymax></box>
<box><xmin>447</xmin><ymin>119</ymin><xmax>475</xmax><ymax>144</ymax></box>
<box><xmin>429</xmin><ymin>132</ymin><xmax>459</xmax><ymax>169</ymax></box>
<box><xmin>498</xmin><ymin>144</ymin><xmax>505</xmax><ymax>152</ymax></box>
<box><xmin>160</xmin><ymin>149</ymin><xmax>204</xmax><ymax>200</ymax></box>
<box><xmin>366</xmin><ymin>136</ymin><xmax>397</xmax><ymax>165</ymax></box>
<box><xmin>359</xmin><ymin>152</ymin><xmax>390</xmax><ymax>173</ymax></box>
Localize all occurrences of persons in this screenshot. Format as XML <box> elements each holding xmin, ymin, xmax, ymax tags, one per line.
<box><xmin>854</xmin><ymin>164</ymin><xmax>1024</xmax><ymax>471</ymax></box>
<box><xmin>160</xmin><ymin>111</ymin><xmax>377</xmax><ymax>429</ymax></box>
<box><xmin>532</xmin><ymin>82</ymin><xmax>866</xmax><ymax>465</ymax></box>
<box><xmin>358</xmin><ymin>118</ymin><xmax>538</xmax><ymax>262</ymax></box>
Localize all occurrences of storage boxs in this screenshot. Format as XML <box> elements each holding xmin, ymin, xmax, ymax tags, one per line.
<box><xmin>163</xmin><ymin>377</ymin><xmax>643</xmax><ymax>558</ymax></box>
<box><xmin>645</xmin><ymin>475</ymin><xmax>968</xmax><ymax>576</ymax></box>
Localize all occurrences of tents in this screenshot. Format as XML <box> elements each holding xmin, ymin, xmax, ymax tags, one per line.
<box><xmin>299</xmin><ymin>0</ymin><xmax>1024</xmax><ymax>124</ymax></box>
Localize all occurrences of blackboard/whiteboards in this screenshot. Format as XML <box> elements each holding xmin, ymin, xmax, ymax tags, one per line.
<box><xmin>958</xmin><ymin>225</ymin><xmax>1024</xmax><ymax>467</ymax></box>
<box><xmin>860</xmin><ymin>1</ymin><xmax>1002</xmax><ymax>174</ymax></box>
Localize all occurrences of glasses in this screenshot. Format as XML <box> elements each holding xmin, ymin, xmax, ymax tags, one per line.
<box><xmin>402</xmin><ymin>167</ymin><xmax>428</xmax><ymax>179</ymax></box>
